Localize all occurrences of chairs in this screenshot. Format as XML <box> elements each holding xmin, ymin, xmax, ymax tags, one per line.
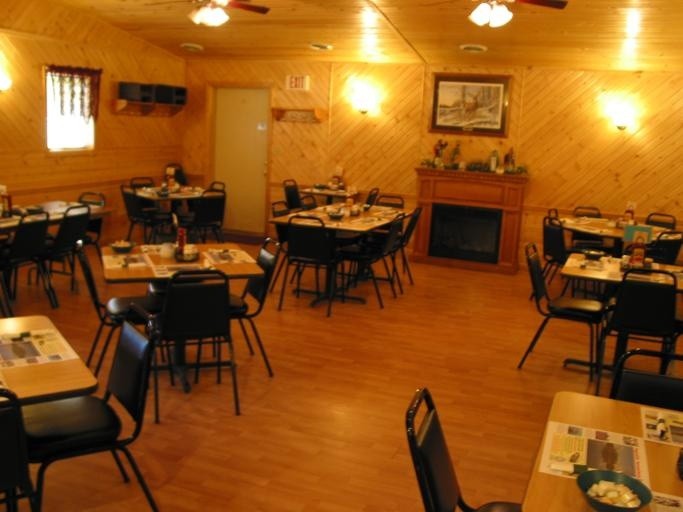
<box><xmin>516</xmin><ymin>203</ymin><xmax>683</xmax><ymax>411</ymax></box>
<box><xmin>275</xmin><ymin>176</ymin><xmax>425</xmax><ymax>319</ymax></box>
<box><xmin>20</xmin><ymin>313</ymin><xmax>160</xmax><ymax>511</ymax></box>
<box><xmin>0</xmin><ymin>162</ymin><xmax>225</xmax><ymax>311</ymax></box>
<box><xmin>148</xmin><ymin>269</ymin><xmax>240</xmax><ymax>424</ymax></box>
<box><xmin>194</xmin><ymin>237</ymin><xmax>280</xmax><ymax>383</ymax></box>
<box><xmin>2</xmin><ymin>389</ymin><xmax>40</xmax><ymax>510</ymax></box>
<box><xmin>75</xmin><ymin>239</ymin><xmax>160</xmax><ymax>378</ymax></box>
<box><xmin>402</xmin><ymin>384</ymin><xmax>523</xmax><ymax>512</ymax></box>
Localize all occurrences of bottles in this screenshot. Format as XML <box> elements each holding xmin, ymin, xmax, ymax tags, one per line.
<box><xmin>141</xmin><ymin>165</ymin><xmax>200</xmax><ymax>197</ymax></box>
<box><xmin>431</xmin><ymin>137</ymin><xmax>515</xmax><ymax>175</ymax></box>
<box><xmin>314</xmin><ymin>175</ymin><xmax>370</xmax><ymax>219</ymax></box>
<box><xmin>617</xmin><ymin>248</ymin><xmax>653</xmax><ymax>273</ymax></box>
<box><xmin>0</xmin><ymin>184</ymin><xmax>43</xmax><ymax>219</ymax></box>
<box><xmin>160</xmin><ymin>228</ymin><xmax>198</xmax><ymax>262</ymax></box>
<box><xmin>605</xmin><ymin>204</ymin><xmax>636</xmax><ymax>230</ymax></box>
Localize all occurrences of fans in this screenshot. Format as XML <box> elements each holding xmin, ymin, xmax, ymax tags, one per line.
<box><xmin>144</xmin><ymin>0</ymin><xmax>271</xmax><ymax>21</ymax></box>
<box><xmin>458</xmin><ymin>0</ymin><xmax>569</xmax><ymax>15</ymax></box>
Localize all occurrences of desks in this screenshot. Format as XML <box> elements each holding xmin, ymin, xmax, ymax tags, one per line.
<box><xmin>0</xmin><ymin>316</ymin><xmax>98</xmax><ymax>405</ymax></box>
<box><xmin>100</xmin><ymin>242</ymin><xmax>266</xmax><ymax>367</ymax></box>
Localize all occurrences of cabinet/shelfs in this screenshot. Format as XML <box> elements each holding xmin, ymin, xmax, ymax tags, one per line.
<box><xmin>114</xmin><ymin>81</ymin><xmax>189</xmax><ymax>118</ymax></box>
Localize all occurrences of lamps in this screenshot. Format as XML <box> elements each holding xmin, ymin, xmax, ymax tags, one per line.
<box><xmin>184</xmin><ymin>4</ymin><xmax>232</xmax><ymax>30</ymax></box>
<box><xmin>464</xmin><ymin>0</ymin><xmax>517</xmax><ymax>29</ymax></box>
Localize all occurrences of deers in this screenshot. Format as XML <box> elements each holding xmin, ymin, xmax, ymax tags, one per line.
<box><xmin>463</xmin><ymin>91</ymin><xmax>480</xmax><ymax>122</ymax></box>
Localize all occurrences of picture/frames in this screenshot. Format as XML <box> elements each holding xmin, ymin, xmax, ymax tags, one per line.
<box><xmin>427</xmin><ymin>70</ymin><xmax>512</xmax><ymax>140</ymax></box>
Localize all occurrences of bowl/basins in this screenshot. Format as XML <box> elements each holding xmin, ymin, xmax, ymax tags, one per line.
<box><xmin>573</xmin><ymin>469</ymin><xmax>652</xmax><ymax>511</ymax></box>
<box><xmin>110</xmin><ymin>240</ymin><xmax>136</xmax><ymax>253</ymax></box>
<box><xmin>580</xmin><ymin>248</ymin><xmax>606</xmax><ymax>259</ymax></box>
